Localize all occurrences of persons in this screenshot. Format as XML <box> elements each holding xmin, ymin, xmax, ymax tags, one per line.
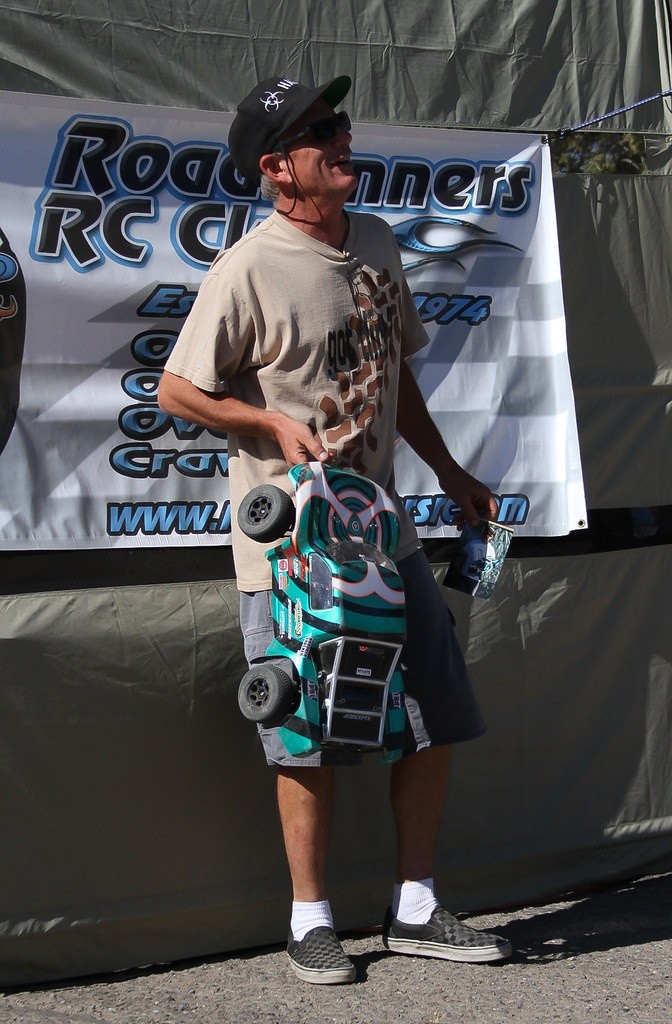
<box><xmin>156</xmin><ymin>70</ymin><xmax>513</xmax><ymax>985</ymax></box>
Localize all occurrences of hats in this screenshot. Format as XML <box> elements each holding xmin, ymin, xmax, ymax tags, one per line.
<box><xmin>228</xmin><ymin>75</ymin><xmax>352</xmax><ymax>186</ymax></box>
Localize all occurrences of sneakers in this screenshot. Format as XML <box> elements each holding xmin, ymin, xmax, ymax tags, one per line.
<box><xmin>382</xmin><ymin>903</ymin><xmax>513</xmax><ymax>962</ymax></box>
<box><xmin>286</xmin><ymin>926</ymin><xmax>357</xmax><ymax>984</ymax></box>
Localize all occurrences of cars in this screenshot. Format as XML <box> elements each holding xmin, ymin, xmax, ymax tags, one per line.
<box><xmin>234</xmin><ymin>461</ymin><xmax>419</xmax><ymax>767</ymax></box>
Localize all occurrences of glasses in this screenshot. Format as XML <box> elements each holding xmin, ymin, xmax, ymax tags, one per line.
<box><xmin>272</xmin><ymin>111</ymin><xmax>351</xmax><ymax>154</ymax></box>
